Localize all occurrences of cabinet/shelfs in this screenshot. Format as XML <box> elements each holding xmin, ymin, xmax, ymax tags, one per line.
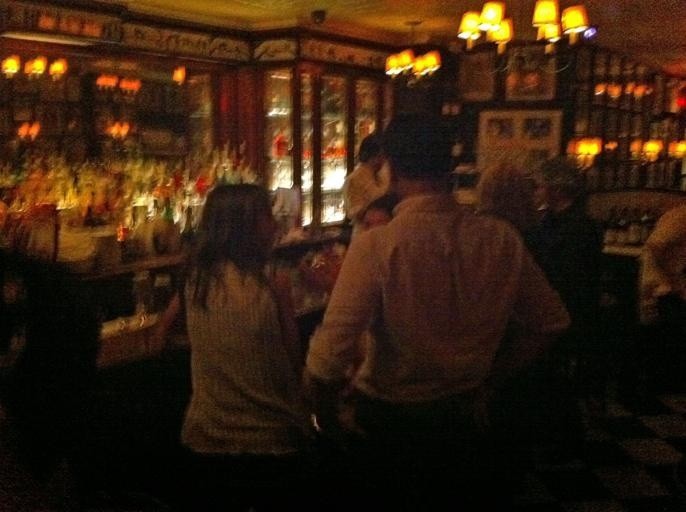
<box><xmin>255</xmin><ymin>31</ymin><xmax>394</xmax><ymax>244</ymax></box>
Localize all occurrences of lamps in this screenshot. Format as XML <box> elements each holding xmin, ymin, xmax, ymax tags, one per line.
<box><xmin>453</xmin><ymin>1</ymin><xmax>593</xmax><ymax>54</ymax></box>
<box><xmin>379</xmin><ymin>18</ymin><xmax>442</xmax><ymax>77</ymax></box>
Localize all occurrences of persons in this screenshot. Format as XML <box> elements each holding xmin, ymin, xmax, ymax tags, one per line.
<box><xmin>2</xmin><ymin>107</ymin><xmax>686</xmax><ymax>511</ymax></box>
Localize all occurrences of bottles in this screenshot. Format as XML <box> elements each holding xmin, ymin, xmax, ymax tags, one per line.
<box><xmin>604</xmin><ymin>203</ymin><xmax>655</xmax><ymax>245</ymax></box>
<box><xmin>3</xmin><ymin>198</ymin><xmax>59</xmax><ymax>261</ymax></box>
<box><xmin>76</xmin><ymin>192</ymin><xmax>197</xmax><ymax>270</ymax></box>
<box><xmin>189</xmin><ymin>140</ymin><xmax>258</xmax><ymax>191</ymax></box>
